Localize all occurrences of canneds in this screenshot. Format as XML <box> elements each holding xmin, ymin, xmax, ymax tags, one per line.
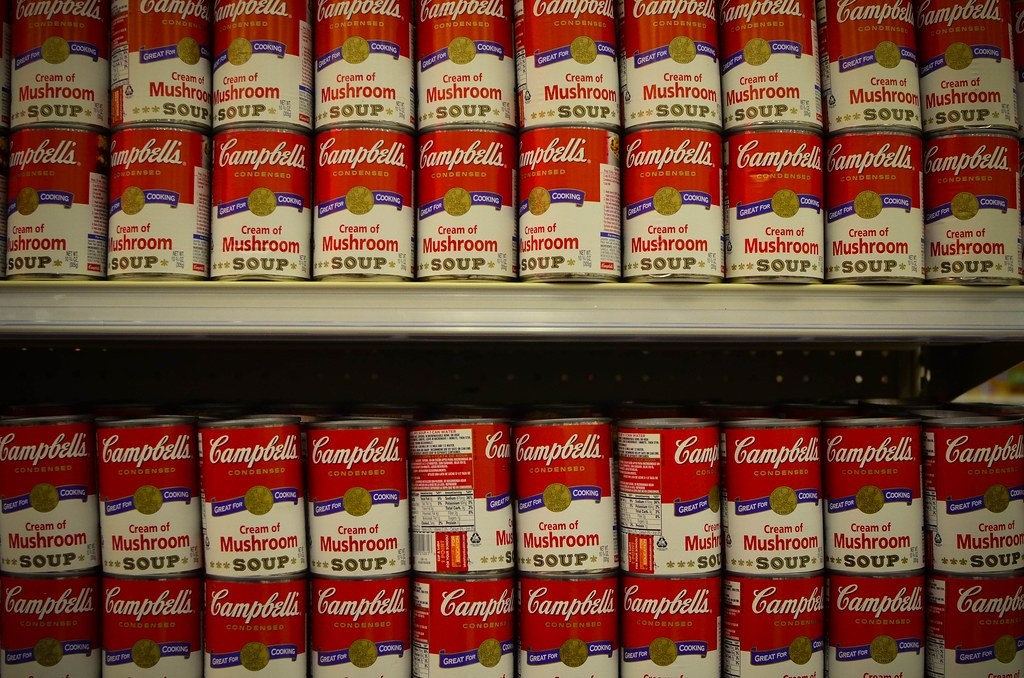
<box><xmin>0</xmin><ymin>2</ymin><xmax>1023</xmax><ymax>288</ymax></box>
<box><xmin>0</xmin><ymin>416</ymin><xmax>1024</xmax><ymax>678</ymax></box>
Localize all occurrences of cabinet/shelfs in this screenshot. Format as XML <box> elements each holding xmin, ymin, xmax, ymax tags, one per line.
<box><xmin>0</xmin><ymin>0</ymin><xmax>1024</xmax><ymax>678</ymax></box>
